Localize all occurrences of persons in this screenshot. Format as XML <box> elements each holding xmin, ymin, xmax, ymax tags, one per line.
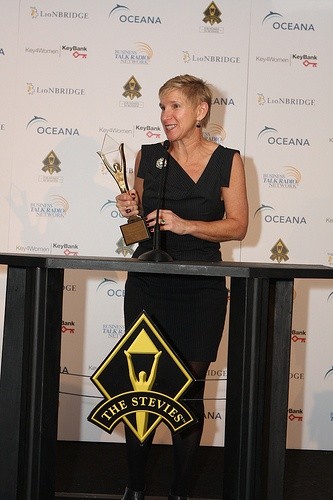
<box><xmin>117</xmin><ymin>75</ymin><xmax>249</xmax><ymax>500</ymax></box>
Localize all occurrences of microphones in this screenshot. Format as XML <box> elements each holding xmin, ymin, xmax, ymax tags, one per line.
<box><xmin>154</xmin><ymin>140</ymin><xmax>171</xmax><ymax>249</ymax></box>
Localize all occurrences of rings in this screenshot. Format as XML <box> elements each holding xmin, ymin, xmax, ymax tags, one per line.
<box><xmin>126</xmin><ymin>207</ymin><xmax>131</xmax><ymax>213</ymax></box>
<box><xmin>161</xmin><ymin>218</ymin><xmax>165</xmax><ymax>224</ymax></box>
